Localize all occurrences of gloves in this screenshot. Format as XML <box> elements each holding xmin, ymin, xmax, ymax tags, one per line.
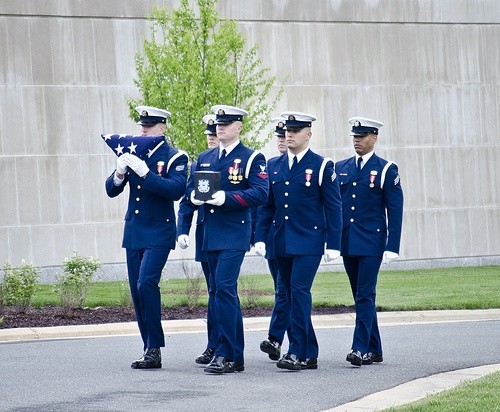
<box><xmin>205</xmin><ymin>190</ymin><xmax>225</xmax><ymax>207</ymax></box>
<box><xmin>177</xmin><ymin>234</ymin><xmax>190</xmax><ymax>249</ymax></box>
<box><xmin>124</xmin><ymin>153</ymin><xmax>150</xmax><ymax>177</ymax></box>
<box><xmin>190</xmin><ymin>189</ymin><xmax>204</xmax><ymax>205</ymax></box>
<box><xmin>382</xmin><ymin>250</ymin><xmax>399</xmax><ymax>265</ymax></box>
<box><xmin>254</xmin><ymin>241</ymin><xmax>267</xmax><ymax>257</ymax></box>
<box><xmin>116</xmin><ymin>152</ymin><xmax>129</xmax><ymax>175</ymax></box>
<box><xmin>324</xmin><ymin>249</ymin><xmax>341</xmax><ymax>263</ymax></box>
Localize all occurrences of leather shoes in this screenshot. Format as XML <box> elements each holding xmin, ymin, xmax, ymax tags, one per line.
<box><xmin>362</xmin><ymin>352</ymin><xmax>383</xmax><ymax>364</ymax></box>
<box><xmin>260</xmin><ymin>339</ymin><xmax>281</xmax><ymax>361</ymax></box>
<box><xmin>300</xmin><ymin>356</ymin><xmax>318</xmax><ymax>370</ymax></box>
<box><xmin>195</xmin><ymin>350</ymin><xmax>215</xmax><ymax>364</ymax></box>
<box><xmin>346</xmin><ymin>349</ymin><xmax>363</xmax><ymax>367</ymax></box>
<box><xmin>139</xmin><ymin>347</ymin><xmax>162</xmax><ymax>369</ymax></box>
<box><xmin>131</xmin><ymin>352</ymin><xmax>146</xmax><ymax>369</ymax></box>
<box><xmin>276</xmin><ymin>353</ymin><xmax>302</xmax><ymax>371</ymax></box>
<box><xmin>204</xmin><ymin>355</ymin><xmax>230</xmax><ymax>374</ymax></box>
<box><xmin>225</xmin><ymin>361</ymin><xmax>245</xmax><ymax>373</ymax></box>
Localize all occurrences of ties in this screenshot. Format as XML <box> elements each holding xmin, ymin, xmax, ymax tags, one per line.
<box><xmin>290</xmin><ymin>156</ymin><xmax>298</xmax><ymax>172</ymax></box>
<box><xmin>356</xmin><ymin>157</ymin><xmax>363</xmax><ymax>170</ymax></box>
<box><xmin>219</xmin><ymin>149</ymin><xmax>227</xmax><ymax>162</ymax></box>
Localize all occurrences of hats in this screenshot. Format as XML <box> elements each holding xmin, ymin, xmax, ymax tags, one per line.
<box><xmin>202</xmin><ymin>115</ymin><xmax>218</xmax><ymax>135</ymax></box>
<box><xmin>348</xmin><ymin>117</ymin><xmax>384</xmax><ymax>136</ymax></box>
<box><xmin>210</xmin><ymin>104</ymin><xmax>248</xmax><ymax>125</ymax></box>
<box><xmin>134</xmin><ymin>106</ymin><xmax>171</xmax><ymax>126</ymax></box>
<box><xmin>281</xmin><ymin>112</ymin><xmax>316</xmax><ymax>130</ymax></box>
<box><xmin>272</xmin><ymin>118</ymin><xmax>287</xmax><ymax>136</ymax></box>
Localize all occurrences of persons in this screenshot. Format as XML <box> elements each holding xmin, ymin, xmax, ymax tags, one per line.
<box><xmin>105</xmin><ymin>106</ymin><xmax>189</xmax><ymax>369</ymax></box>
<box><xmin>250</xmin><ymin>117</ymin><xmax>288</xmax><ymax>362</ymax></box>
<box><xmin>187</xmin><ymin>104</ymin><xmax>269</xmax><ymax>374</ymax></box>
<box><xmin>254</xmin><ymin>111</ymin><xmax>342</xmax><ymax>371</ymax></box>
<box><xmin>176</xmin><ymin>114</ymin><xmax>220</xmax><ymax>364</ymax></box>
<box><xmin>333</xmin><ymin>117</ymin><xmax>404</xmax><ymax>366</ymax></box>
